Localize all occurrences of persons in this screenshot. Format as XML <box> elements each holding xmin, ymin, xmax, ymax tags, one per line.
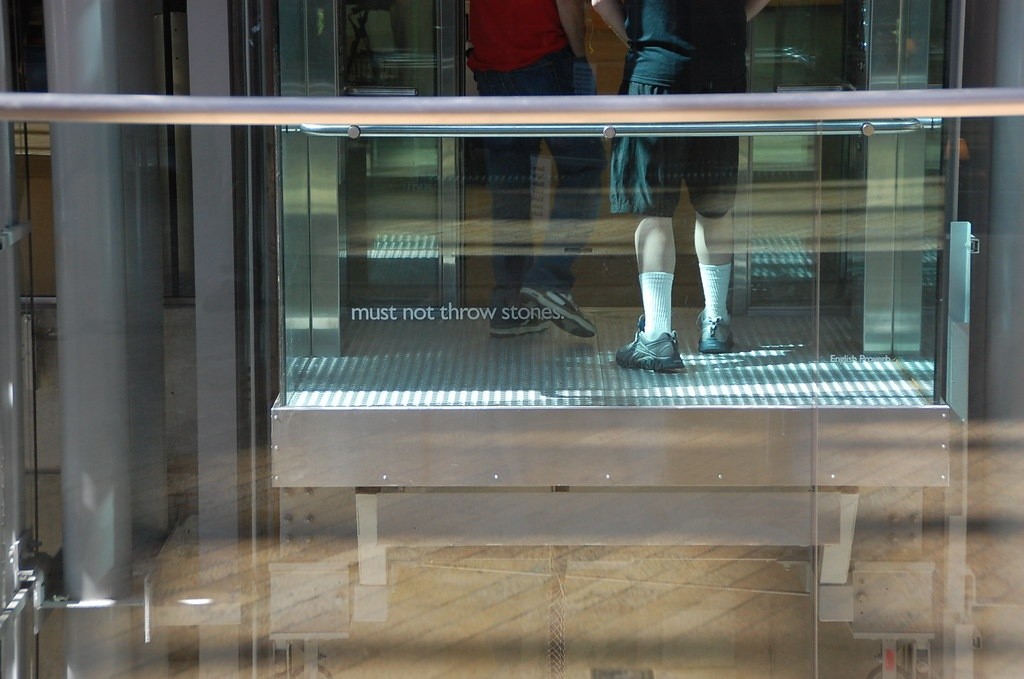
<box><xmin>591</xmin><ymin>0</ymin><xmax>770</xmax><ymax>372</ymax></box>
<box><xmin>464</xmin><ymin>0</ymin><xmax>603</xmax><ymax>337</ymax></box>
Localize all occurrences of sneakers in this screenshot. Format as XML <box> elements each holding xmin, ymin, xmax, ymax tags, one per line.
<box><xmin>615</xmin><ymin>314</ymin><xmax>688</xmax><ymax>373</ymax></box>
<box><xmin>489</xmin><ymin>315</ymin><xmax>551</xmax><ymax>338</ymax></box>
<box><xmin>519</xmin><ymin>283</ymin><xmax>597</xmax><ymax>338</ymax></box>
<box><xmin>696</xmin><ymin>310</ymin><xmax>734</xmax><ymax>353</ymax></box>
